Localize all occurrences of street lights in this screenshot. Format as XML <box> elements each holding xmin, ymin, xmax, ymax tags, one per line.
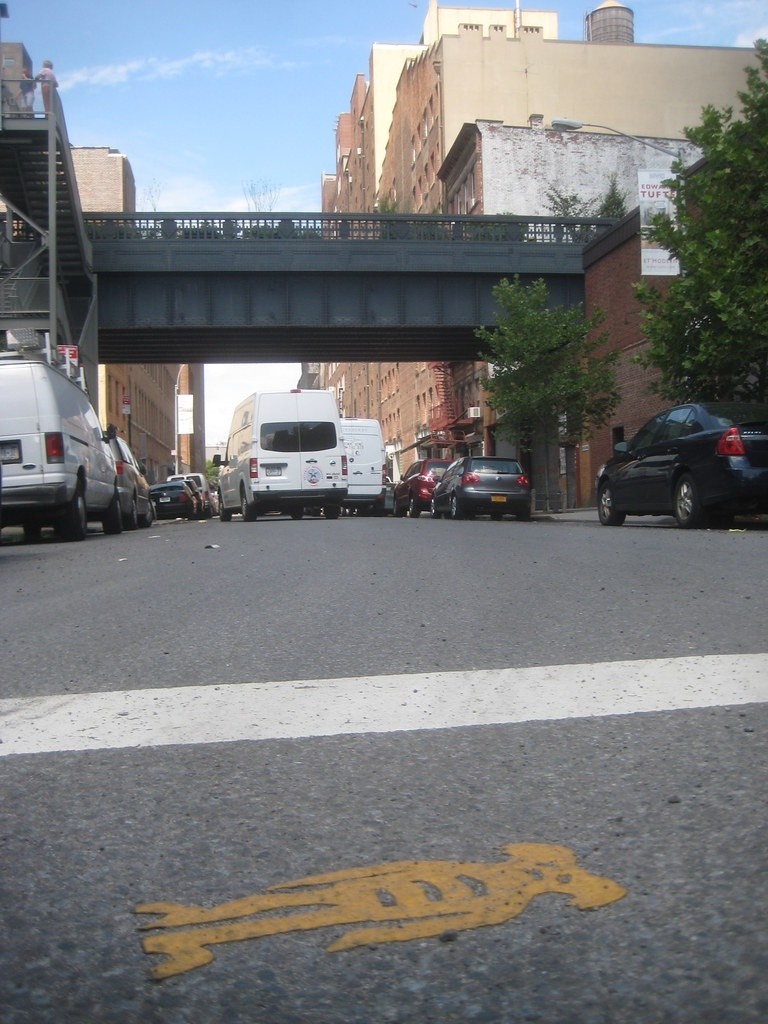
<box><xmin>552</xmin><ymin>119</ymin><xmax>684</xmax><ymax>275</ymax></box>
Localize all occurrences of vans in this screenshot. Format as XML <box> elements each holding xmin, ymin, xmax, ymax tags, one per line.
<box><xmin>166</xmin><ymin>473</ymin><xmax>213</xmax><ymax>519</ymax></box>
<box><xmin>0</xmin><ymin>327</ymin><xmax>123</xmax><ymax>542</ymax></box>
<box><xmin>304</xmin><ymin>417</ymin><xmax>387</xmax><ymax>517</ymax></box>
<box><xmin>212</xmin><ymin>389</ymin><xmax>348</xmax><ymax>523</ymax></box>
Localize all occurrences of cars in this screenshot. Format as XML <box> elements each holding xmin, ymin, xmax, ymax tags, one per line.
<box><xmin>595</xmin><ymin>401</ymin><xmax>768</xmax><ymax>529</ymax></box>
<box><xmin>102</xmin><ymin>431</ymin><xmax>154</xmax><ymax>531</ymax></box>
<box><xmin>430</xmin><ymin>456</ymin><xmax>531</xmax><ymax>521</ymax></box>
<box><xmin>150</xmin><ymin>479</ymin><xmax>205</xmax><ymax>520</ymax></box>
<box><xmin>393</xmin><ymin>458</ymin><xmax>457</xmax><ymax>518</ymax></box>
